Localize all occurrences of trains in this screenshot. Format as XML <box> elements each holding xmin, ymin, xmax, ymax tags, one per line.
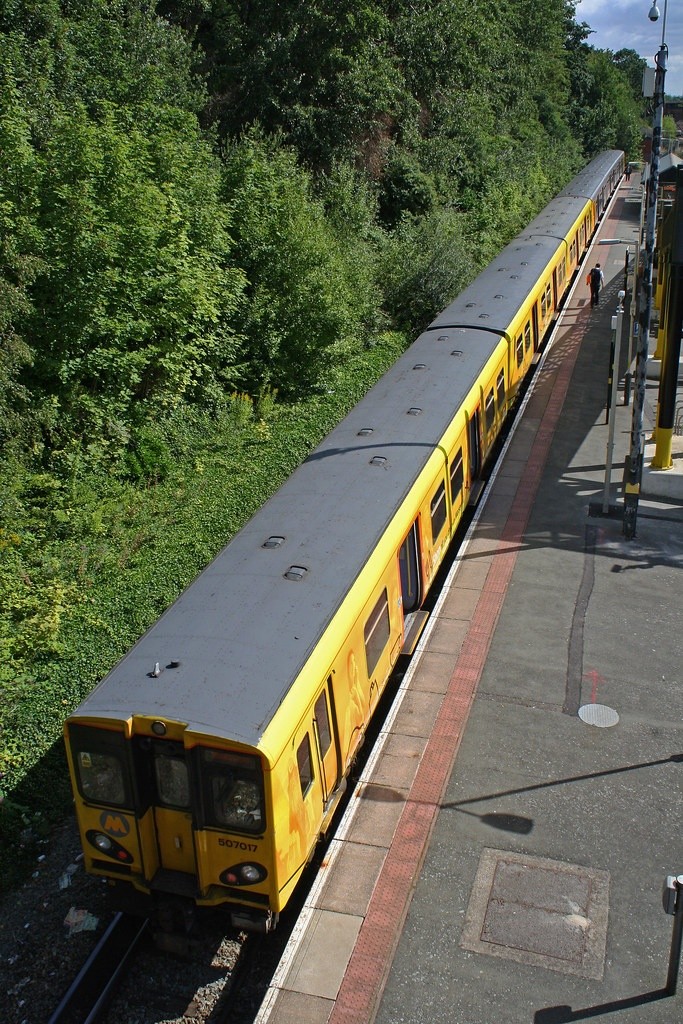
<box><xmin>63</xmin><ymin>150</ymin><xmax>625</xmax><ymax>934</ymax></box>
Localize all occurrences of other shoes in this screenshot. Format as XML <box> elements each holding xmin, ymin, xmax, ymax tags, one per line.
<box><xmin>595</xmin><ymin>302</ymin><xmax>598</xmax><ymax>306</ymax></box>
<box><xmin>590</xmin><ymin>306</ymin><xmax>593</xmax><ymax>309</ymax></box>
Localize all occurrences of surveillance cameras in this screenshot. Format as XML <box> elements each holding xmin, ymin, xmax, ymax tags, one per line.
<box><xmin>648</xmin><ymin>6</ymin><xmax>660</xmax><ymax>21</ymax></box>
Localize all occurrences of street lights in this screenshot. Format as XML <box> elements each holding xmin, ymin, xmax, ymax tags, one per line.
<box><xmin>599</xmin><ymin>239</ymin><xmax>639</xmax><ymax>370</ymax></box>
<box><xmin>619</xmin><ymin>186</ymin><xmax>644</xmax><ymax>231</ymax></box>
<box><xmin>629</xmin><ymin>162</ymin><xmax>648</xmax><ymax>191</ymax></box>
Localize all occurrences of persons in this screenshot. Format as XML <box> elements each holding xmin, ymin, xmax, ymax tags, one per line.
<box><xmin>586</xmin><ymin>263</ymin><xmax>605</xmax><ymax>309</ymax></box>
<box><xmin>623</xmin><ymin>164</ymin><xmax>633</xmax><ymax>180</ymax></box>
